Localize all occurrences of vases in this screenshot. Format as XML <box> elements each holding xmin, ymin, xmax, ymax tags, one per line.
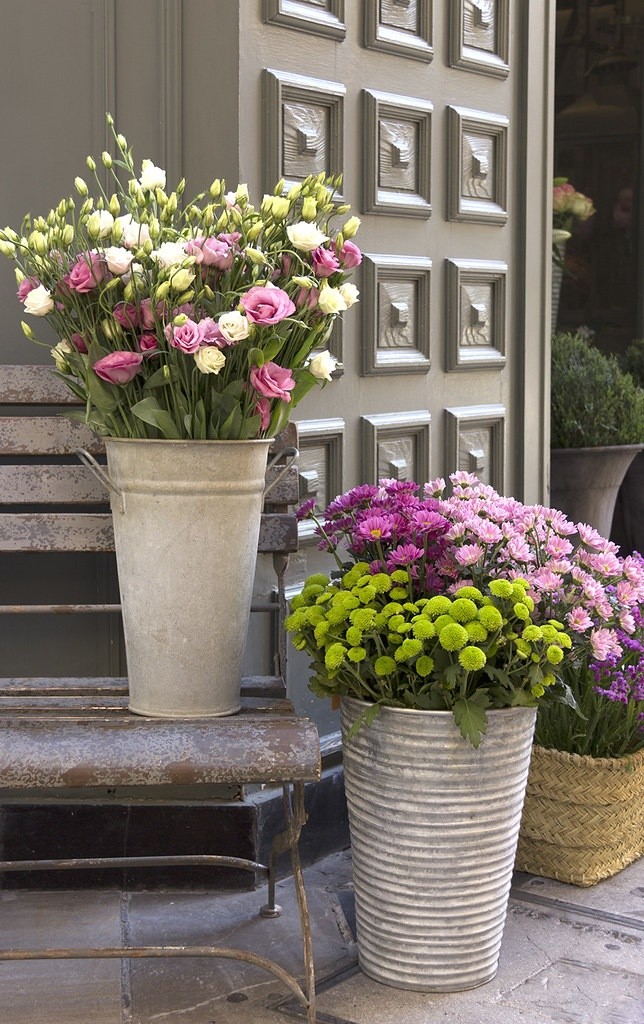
<box><xmin>338</xmin><ymin>695</ymin><xmax>536</xmax><ymax>995</ymax></box>
<box><xmin>552</xmin><ymin>230</ymin><xmax>568</xmax><ymax>336</ymax></box>
<box><xmin>78</xmin><ymin>438</ymin><xmax>300</xmax><ymax>718</ymax></box>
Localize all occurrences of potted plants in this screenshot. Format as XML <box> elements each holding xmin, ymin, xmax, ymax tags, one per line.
<box><xmin>615</xmin><ymin>339</ymin><xmax>644</xmax><ymax>560</ymax></box>
<box><xmin>549</xmin><ymin>333</ymin><xmax>644</xmax><ymax>541</ymax></box>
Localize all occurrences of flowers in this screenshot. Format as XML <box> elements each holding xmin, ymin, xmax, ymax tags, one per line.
<box><xmin>552</xmin><ymin>177</ymin><xmax>597</xmax><ymax>284</ymax></box>
<box><xmin>0</xmin><ymin>112</ymin><xmax>362</xmax><ymax>440</ymax></box>
<box><xmin>288</xmin><ymin>480</ymin><xmax>581</xmax><ymax>753</ymax></box>
<box><xmin>532</xmin><ymin>522</ymin><xmax>644</xmax><ymax>758</ymax></box>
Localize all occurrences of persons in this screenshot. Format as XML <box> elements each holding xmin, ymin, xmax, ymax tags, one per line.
<box><xmin>598</xmin><ymin>184</ymin><xmax>634</xmax><ymax>314</ymax></box>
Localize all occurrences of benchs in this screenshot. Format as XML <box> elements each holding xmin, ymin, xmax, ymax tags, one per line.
<box><xmin>0</xmin><ymin>366</ymin><xmax>322</xmax><ymax>1024</ymax></box>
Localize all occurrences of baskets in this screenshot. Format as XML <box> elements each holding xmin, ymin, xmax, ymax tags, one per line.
<box><xmin>514</xmin><ymin>745</ymin><xmax>644</xmax><ymax>887</ymax></box>
